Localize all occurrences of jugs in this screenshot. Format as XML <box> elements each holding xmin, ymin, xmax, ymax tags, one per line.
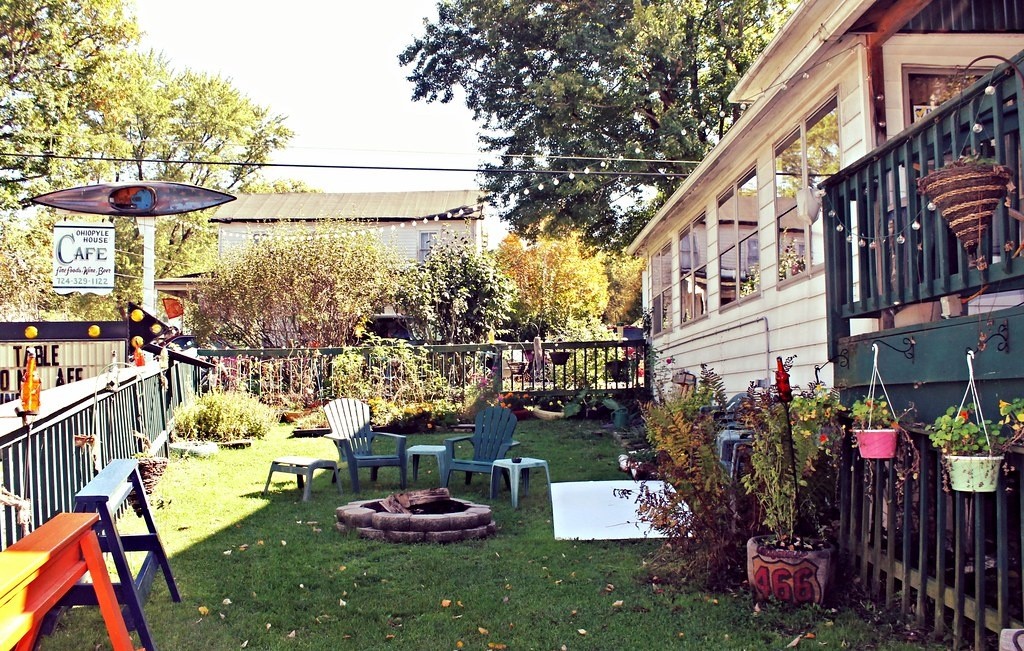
<box><xmin>611</xmin><ymin>407</ymin><xmax>640</xmax><ymax>430</ymax></box>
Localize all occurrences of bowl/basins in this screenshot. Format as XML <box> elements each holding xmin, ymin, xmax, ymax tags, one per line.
<box><xmin>512</xmin><ymin>458</ymin><xmax>522</xmax><ymax>463</ymax></box>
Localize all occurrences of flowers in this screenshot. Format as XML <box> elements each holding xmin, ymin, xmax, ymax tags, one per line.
<box><xmin>924</xmin><ymin>397</ymin><xmax>1024</xmax><ymax>457</ymax></box>
<box><xmin>848</xmin><ymin>395</ymin><xmax>899</xmax><ymax>430</ymax></box>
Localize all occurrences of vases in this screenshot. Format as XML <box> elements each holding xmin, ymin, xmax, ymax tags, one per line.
<box><xmin>945</xmin><ymin>456</ymin><xmax>1004</xmax><ymax>492</ymax></box>
<box><xmin>854</xmin><ymin>429</ymin><xmax>897</xmax><ymax>458</ymax></box>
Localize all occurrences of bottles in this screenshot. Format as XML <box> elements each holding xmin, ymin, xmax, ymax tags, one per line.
<box><xmin>134</xmin><ymin>337</ymin><xmax>145</xmax><ymax>366</ymax></box>
<box><xmin>20</xmin><ymin>347</ymin><xmax>41</xmax><ymax>414</ymax></box>
<box><xmin>776</xmin><ymin>357</ymin><xmax>792</xmax><ymax>401</ymax></box>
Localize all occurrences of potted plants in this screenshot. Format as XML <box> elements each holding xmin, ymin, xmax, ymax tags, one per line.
<box><xmin>732</xmin><ymin>383</ymin><xmax>839</xmax><ymax>615</ymax></box>
<box><xmin>919</xmin><ymin>153</ymin><xmax>1014</xmax><ymax>251</ymax></box>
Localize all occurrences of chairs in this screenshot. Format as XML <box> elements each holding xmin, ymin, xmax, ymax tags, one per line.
<box><xmin>442</xmin><ymin>405</ymin><xmax>521</xmax><ymax>491</ymax></box>
<box><xmin>323</xmin><ymin>398</ymin><xmax>406</xmax><ymax>496</ymax></box>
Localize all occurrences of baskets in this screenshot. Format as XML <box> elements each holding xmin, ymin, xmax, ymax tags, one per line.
<box><xmin>507</xmin><ymin>341</ymin><xmax>530</xmax><ymax>375</ymax></box>
<box><xmin>604</xmin><ymin>360</ymin><xmax>623</xmax><ymax>380</ymax></box>
<box><xmin>549</xmin><ymin>338</ymin><xmax>571</xmax><ymax>365</ymax></box>
<box><xmin>917</xmin><ymin>164</ymin><xmax>1013</xmax><ymax>254</ymax></box>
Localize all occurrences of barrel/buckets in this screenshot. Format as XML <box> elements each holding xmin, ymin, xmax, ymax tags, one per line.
<box><xmin>604</xmin><ymin>360</ymin><xmax>640</xmax><ymax>381</ymax></box>
<box><xmin>506</xmin><ymin>341</ymin><xmax>529</xmax><ymax>374</ymax></box>
<box><xmin>549</xmin><ymin>339</ymin><xmax>571</xmax><ymax>365</ymax></box>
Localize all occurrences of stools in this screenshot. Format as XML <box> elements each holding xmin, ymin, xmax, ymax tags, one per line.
<box><xmin>0</xmin><ymin>513</ymin><xmax>136</xmax><ymax>651</ymax></box>
<box><xmin>38</xmin><ymin>459</ymin><xmax>181</xmax><ymax>651</ymax></box>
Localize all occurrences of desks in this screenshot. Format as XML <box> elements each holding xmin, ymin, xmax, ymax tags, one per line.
<box><xmin>489</xmin><ymin>457</ymin><xmax>551</xmax><ymax>508</ymax></box>
<box><xmin>407</xmin><ymin>445</ymin><xmax>447</xmax><ymax>488</ymax></box>
<box><xmin>262</xmin><ymin>456</ymin><xmax>344</xmax><ymax>501</ymax></box>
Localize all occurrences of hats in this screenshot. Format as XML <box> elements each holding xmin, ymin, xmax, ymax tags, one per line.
<box><xmin>525</xmin><ymin>340</ymin><xmax>529</xmax><ymax>342</ymax></box>
